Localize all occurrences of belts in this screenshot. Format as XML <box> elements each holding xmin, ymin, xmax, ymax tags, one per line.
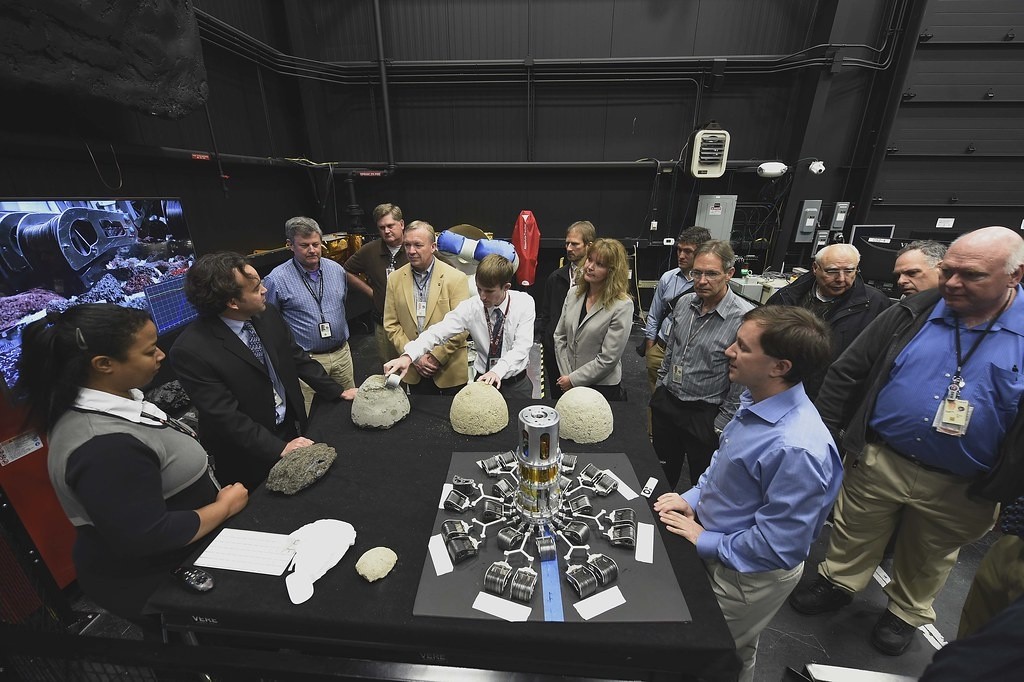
<box><xmin>661</xmin><ymin>383</ymin><xmax>720</xmax><ymax>409</ymax></box>
<box><xmin>303</xmin><ymin>345</ymin><xmax>342</xmax><ymax>354</ymax></box>
<box><xmin>655</xmin><ymin>335</ymin><xmax>667</xmax><ymax>351</ymax></box>
<box><xmin>872</xmin><ymin>435</ymin><xmax>957</xmax><ymax>476</ymax></box>
<box><xmin>477</xmin><ymin>370</ymin><xmax>526</xmax><ymax>385</ymax></box>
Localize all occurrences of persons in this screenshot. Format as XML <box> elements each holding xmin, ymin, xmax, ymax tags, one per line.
<box><xmin>648</xmin><ymin>240</ymin><xmax>755</xmax><ymax>493</ymax></box>
<box><xmin>8</xmin><ymin>303</ymin><xmax>248</xmax><ymax>682</ymax></box>
<box><xmin>792</xmin><ymin>222</ymin><xmax>1024</xmax><ymax>657</ymax></box>
<box><xmin>766</xmin><ymin>243</ymin><xmax>892</xmax><ymax>403</ymax></box>
<box><xmin>553</xmin><ymin>237</ymin><xmax>635</xmax><ymax>402</ymax></box>
<box><xmin>263</xmin><ymin>216</ymin><xmax>357</xmax><ymax>419</ymax></box>
<box><xmin>646</xmin><ymin>225</ymin><xmax>711</xmax><ymax>443</ymax></box>
<box><xmin>653</xmin><ymin>304</ymin><xmax>844</xmax><ymax>682</ymax></box>
<box><xmin>383</xmin><ymin>253</ymin><xmax>536</xmax><ymax>399</ymax></box>
<box><xmin>542</xmin><ymin>221</ymin><xmax>596</xmax><ymax>399</ymax></box>
<box><xmin>169</xmin><ymin>251</ymin><xmax>360</xmax><ymax>494</ymax></box>
<box><xmin>345</xmin><ymin>203</ymin><xmax>454</xmax><ymax>396</ymax></box>
<box><xmin>894</xmin><ymin>239</ymin><xmax>951</xmax><ymax>298</ymax></box>
<box><xmin>383</xmin><ymin>219</ymin><xmax>470</xmax><ymax>396</ymax></box>
<box><xmin>916</xmin><ymin>533</ymin><xmax>1024</xmax><ymax>682</ymax></box>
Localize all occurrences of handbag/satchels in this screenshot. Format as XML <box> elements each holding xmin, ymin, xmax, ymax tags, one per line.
<box><xmin>636</xmin><ymin>334</ymin><xmax>657</xmax><ymax>357</ymax></box>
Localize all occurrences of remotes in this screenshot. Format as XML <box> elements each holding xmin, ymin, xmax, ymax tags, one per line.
<box><xmin>171</xmin><ymin>567</ymin><xmax>214</xmax><ymax>591</ymax></box>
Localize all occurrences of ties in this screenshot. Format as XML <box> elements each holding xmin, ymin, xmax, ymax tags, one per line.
<box><xmin>485</xmin><ymin>308</ymin><xmax>505</xmax><ymax>386</ymax></box>
<box><xmin>240</xmin><ymin>320</ymin><xmax>264</xmax><ymax>367</ymax></box>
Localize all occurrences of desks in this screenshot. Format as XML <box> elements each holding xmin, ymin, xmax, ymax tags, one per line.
<box><xmin>731</xmin><ymin>275</ymin><xmax>807</xmax><ymax>303</ymax></box>
<box><xmin>153</xmin><ymin>400</ymin><xmax>737</xmax><ymax>682</ymax></box>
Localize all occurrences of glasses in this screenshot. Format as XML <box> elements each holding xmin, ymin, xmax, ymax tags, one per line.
<box><xmin>816</xmin><ymin>261</ymin><xmax>861</xmax><ymax>277</ymax></box>
<box><xmin>689</xmin><ymin>270</ymin><xmax>727</xmax><ymax>280</ymax></box>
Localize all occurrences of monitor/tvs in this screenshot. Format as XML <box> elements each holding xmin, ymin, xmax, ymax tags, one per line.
<box><xmin>0</xmin><ymin>196</ymin><xmax>200</xmax><ymax>401</ymax></box>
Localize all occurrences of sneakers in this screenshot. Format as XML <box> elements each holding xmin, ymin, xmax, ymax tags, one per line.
<box><xmin>873</xmin><ymin>607</ymin><xmax>916</xmax><ymax>656</ymax></box>
<box><xmin>792</xmin><ymin>576</ymin><xmax>852</xmax><ymax>615</ymax></box>
